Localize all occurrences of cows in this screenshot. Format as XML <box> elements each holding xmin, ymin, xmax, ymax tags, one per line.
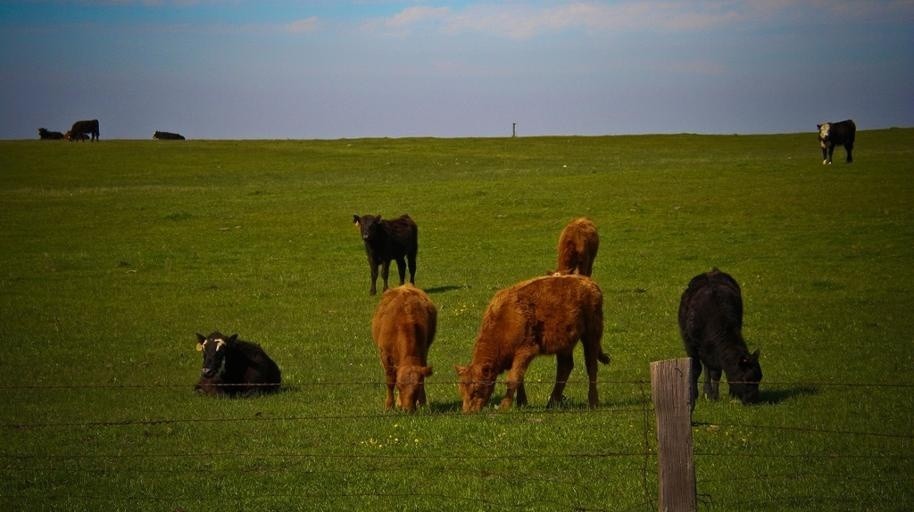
<box><xmin>192</xmin><ymin>331</ymin><xmax>281</xmax><ymax>401</ymax></box>
<box><xmin>678</xmin><ymin>267</ymin><xmax>762</xmax><ymax>426</ymax></box>
<box><xmin>453</xmin><ymin>276</ymin><xmax>612</xmax><ymax>414</ymax></box>
<box><xmin>817</xmin><ymin>119</ymin><xmax>856</xmax><ymax>166</ymax></box>
<box><xmin>38</xmin><ymin>120</ymin><xmax>99</xmax><ymax>143</ymax></box>
<box><xmin>152</xmin><ymin>130</ymin><xmax>186</xmax><ymax>140</ymax></box>
<box><xmin>547</xmin><ymin>217</ymin><xmax>598</xmax><ymax>277</ymax></box>
<box><xmin>353</xmin><ymin>215</ymin><xmax>417</xmax><ymax>295</ymax></box>
<box><xmin>370</xmin><ymin>282</ymin><xmax>436</xmax><ymax>415</ymax></box>
